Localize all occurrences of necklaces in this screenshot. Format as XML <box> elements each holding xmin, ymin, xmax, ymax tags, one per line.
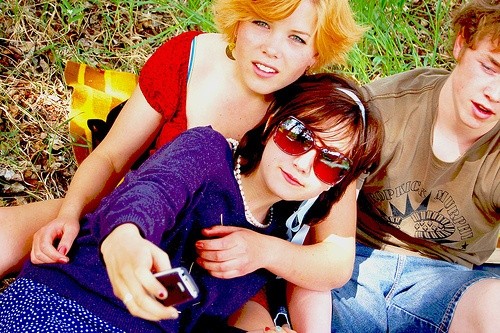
<box><xmin>226</xmin><ymin>137</ymin><xmax>274</xmax><ymax>228</ymax></box>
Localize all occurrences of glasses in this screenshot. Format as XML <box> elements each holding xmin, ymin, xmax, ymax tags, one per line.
<box><xmin>273</xmin><ymin>115</ymin><xmax>353</xmax><ymax>186</ymax></box>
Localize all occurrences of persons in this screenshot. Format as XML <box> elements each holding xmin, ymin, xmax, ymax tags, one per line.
<box><xmin>0</xmin><ymin>0</ymin><xmax>368</xmax><ymax>291</ymax></box>
<box><xmin>0</xmin><ymin>73</ymin><xmax>386</xmax><ymax>333</ymax></box>
<box><xmin>268</xmin><ymin>0</ymin><xmax>500</xmax><ymax>333</ymax></box>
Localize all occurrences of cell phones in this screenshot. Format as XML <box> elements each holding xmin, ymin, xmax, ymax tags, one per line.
<box><xmin>153</xmin><ymin>267</ymin><xmax>199</xmax><ymax>306</ymax></box>
